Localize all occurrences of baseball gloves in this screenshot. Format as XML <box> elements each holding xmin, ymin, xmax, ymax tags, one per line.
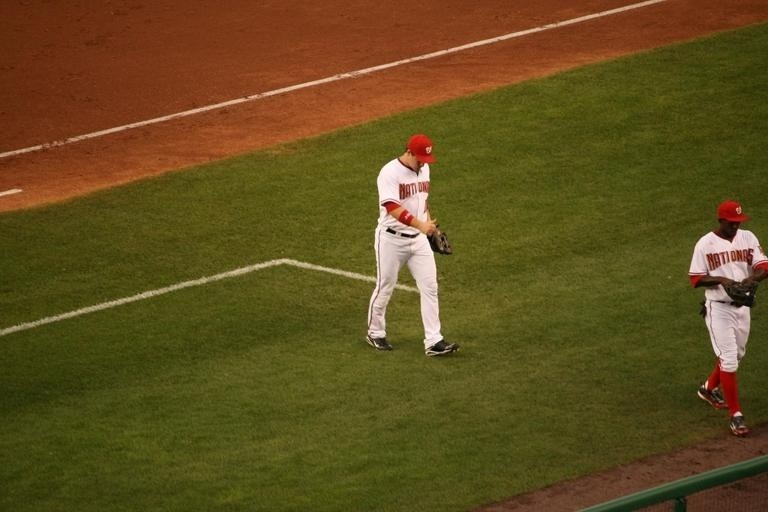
<box><xmin>721</xmin><ymin>280</ymin><xmax>759</xmax><ymax>308</ymax></box>
<box><xmin>428</xmin><ymin>218</ymin><xmax>453</xmax><ymax>254</ymax></box>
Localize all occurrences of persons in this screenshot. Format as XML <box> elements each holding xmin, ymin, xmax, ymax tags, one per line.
<box><xmin>687</xmin><ymin>200</ymin><xmax>767</xmax><ymax>436</ymax></box>
<box><xmin>365</xmin><ymin>131</ymin><xmax>460</xmax><ymax>359</ymax></box>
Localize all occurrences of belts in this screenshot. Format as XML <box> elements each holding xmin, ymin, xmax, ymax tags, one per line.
<box><xmin>388</xmin><ymin>228</ymin><xmax>420</xmax><ymax>238</ymax></box>
<box><xmin>716</xmin><ymin>301</ymin><xmax>737</xmax><ymax>305</ymax></box>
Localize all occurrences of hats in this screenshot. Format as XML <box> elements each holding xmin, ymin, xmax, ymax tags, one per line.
<box><xmin>718</xmin><ymin>201</ymin><xmax>751</xmax><ymax>223</ymax></box>
<box><xmin>407</xmin><ymin>135</ymin><xmax>437</xmax><ymax>164</ymax></box>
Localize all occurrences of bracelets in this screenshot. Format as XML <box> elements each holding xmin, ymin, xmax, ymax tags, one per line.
<box><xmin>398</xmin><ymin>209</ymin><xmax>414</xmax><ymax>226</ymax></box>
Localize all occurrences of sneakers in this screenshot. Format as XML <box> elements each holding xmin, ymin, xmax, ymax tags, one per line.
<box><xmin>728</xmin><ymin>411</ymin><xmax>749</xmax><ymax>436</ymax></box>
<box><xmin>698</xmin><ymin>381</ymin><xmax>727</xmax><ymax>409</ymax></box>
<box><xmin>425</xmin><ymin>341</ymin><xmax>459</xmax><ymax>357</ymax></box>
<box><xmin>365</xmin><ymin>335</ymin><xmax>393</xmax><ymax>350</ymax></box>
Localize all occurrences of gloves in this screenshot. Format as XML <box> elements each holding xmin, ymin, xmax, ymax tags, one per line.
<box><xmin>700</xmin><ymin>300</ymin><xmax>706</xmax><ymax>316</ymax></box>
